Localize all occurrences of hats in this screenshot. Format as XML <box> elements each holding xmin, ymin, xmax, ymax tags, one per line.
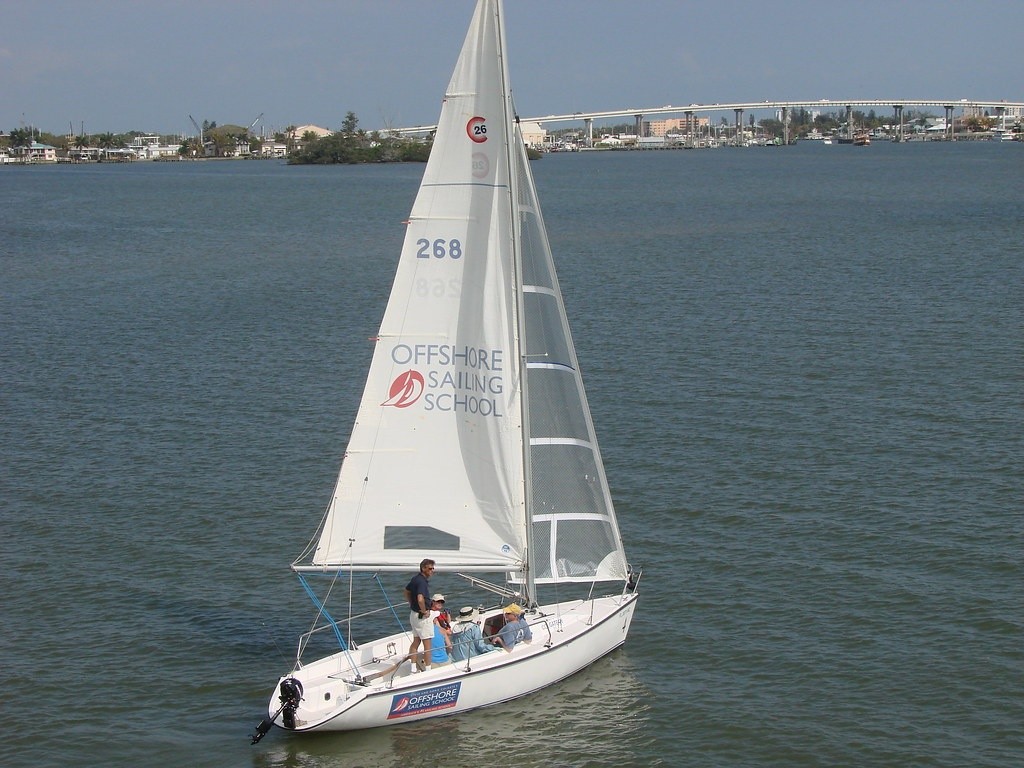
<box><xmin>503</xmin><ymin>604</ymin><xmax>521</xmax><ymax>615</ymax></box>
<box><xmin>430</xmin><ymin>610</ymin><xmax>440</xmax><ymax>620</ymax></box>
<box><xmin>432</xmin><ymin>594</ymin><xmax>445</xmax><ymax>602</ymax></box>
<box><xmin>455</xmin><ymin>607</ymin><xmax>479</xmax><ymax>621</ymax></box>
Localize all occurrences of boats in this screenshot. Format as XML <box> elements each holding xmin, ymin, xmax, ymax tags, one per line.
<box><xmin>804</xmin><ymin>124</ymin><xmax>888</xmax><ymax>146</ymax></box>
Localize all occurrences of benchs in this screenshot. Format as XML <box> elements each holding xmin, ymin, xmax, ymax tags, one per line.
<box><xmin>331</xmin><ymin>646</ymin><xmax>424</xmax><ymax>686</ymax></box>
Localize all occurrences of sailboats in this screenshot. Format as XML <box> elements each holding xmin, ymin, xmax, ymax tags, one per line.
<box><xmin>246</xmin><ymin>0</ymin><xmax>641</xmax><ymax>748</ymax></box>
<box><xmin>533</xmin><ymin>122</ymin><xmax>784</xmax><ymax>156</ymax></box>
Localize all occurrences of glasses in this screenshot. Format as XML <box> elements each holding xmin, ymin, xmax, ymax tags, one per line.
<box><xmin>437</xmin><ymin>601</ymin><xmax>444</xmax><ymax>603</ymax></box>
<box><xmin>425</xmin><ymin>568</ymin><xmax>434</xmax><ymax>570</ymax></box>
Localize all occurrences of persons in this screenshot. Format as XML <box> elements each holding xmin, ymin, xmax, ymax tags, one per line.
<box><xmin>451</xmin><ymin>607</ymin><xmax>503</xmax><ymax>662</ymax></box>
<box><xmin>492</xmin><ymin>603</ymin><xmax>532</xmax><ymax>653</ymax></box>
<box><xmin>404</xmin><ymin>559</ymin><xmax>453</xmax><ymax>676</ymax></box>
